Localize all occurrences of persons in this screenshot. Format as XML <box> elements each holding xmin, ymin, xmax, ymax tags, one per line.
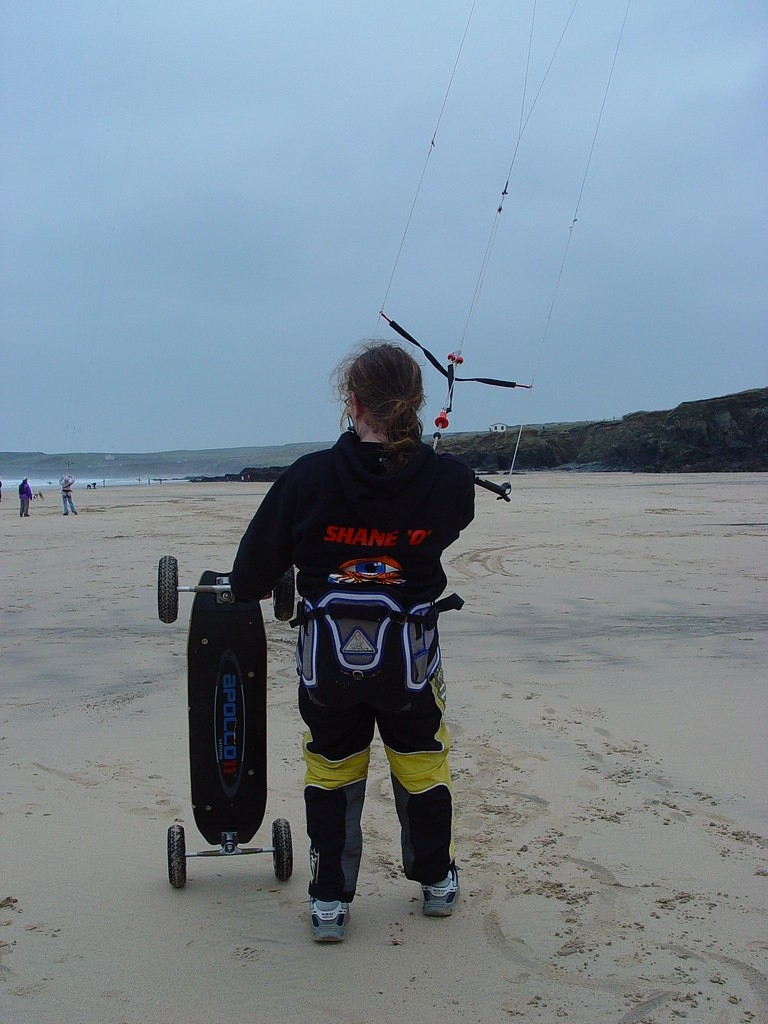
<box><xmin>0</xmin><ymin>481</ymin><xmax>4</xmax><ymax>502</ymax></box>
<box><xmin>59</xmin><ymin>475</ymin><xmax>78</xmax><ymax>516</ymax></box>
<box><xmin>18</xmin><ymin>476</ymin><xmax>32</xmax><ymax>517</ymax></box>
<box><xmin>229</xmin><ymin>344</ymin><xmax>475</xmax><ymax>942</ymax></box>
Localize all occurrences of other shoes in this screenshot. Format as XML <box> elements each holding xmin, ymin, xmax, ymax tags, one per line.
<box><xmin>74</xmin><ymin>511</ymin><xmax>78</xmax><ymax>515</ymax></box>
<box><xmin>63</xmin><ymin>512</ymin><xmax>68</xmax><ymax>515</ymax></box>
<box><xmin>20</xmin><ymin>513</ymin><xmax>22</xmax><ymax>517</ymax></box>
<box><xmin>24</xmin><ymin>513</ymin><xmax>30</xmax><ymax>517</ymax></box>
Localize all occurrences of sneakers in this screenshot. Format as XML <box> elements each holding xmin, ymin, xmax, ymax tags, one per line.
<box><xmin>308</xmin><ymin>895</ymin><xmax>351</xmax><ymax>944</ymax></box>
<box><xmin>421</xmin><ymin>858</ymin><xmax>459</xmax><ymax>917</ymax></box>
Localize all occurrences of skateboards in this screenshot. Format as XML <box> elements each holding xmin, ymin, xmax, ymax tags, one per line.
<box><xmin>157</xmin><ymin>554</ymin><xmax>294</xmax><ymax>888</ymax></box>
